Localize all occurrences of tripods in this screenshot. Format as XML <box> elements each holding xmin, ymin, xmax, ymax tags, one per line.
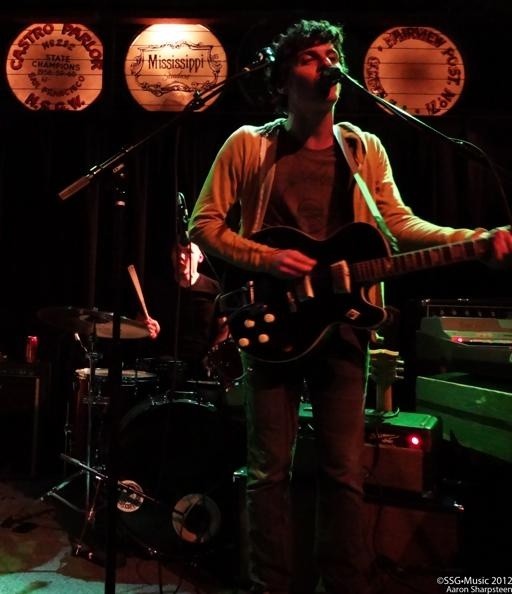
<box><xmin>7</xmin><ymin>318</ymin><xmax>155</xmax><ymax>559</ymax></box>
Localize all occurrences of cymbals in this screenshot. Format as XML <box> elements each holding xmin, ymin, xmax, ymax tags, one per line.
<box><xmin>38</xmin><ymin>306</ymin><xmax>150</xmax><ymax>340</ymax></box>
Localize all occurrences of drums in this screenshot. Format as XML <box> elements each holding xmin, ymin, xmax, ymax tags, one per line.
<box><xmin>74</xmin><ymin>368</ymin><xmax>157</xmax><ymax>406</ymax></box>
<box><xmin>96</xmin><ymin>390</ymin><xmax>248</xmax><ymax>559</ymax></box>
<box><xmin>207</xmin><ymin>336</ymin><xmax>252</xmax><ymax>387</ymax></box>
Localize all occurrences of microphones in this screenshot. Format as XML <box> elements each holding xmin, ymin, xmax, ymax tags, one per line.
<box><xmin>318</xmin><ymin>66</ymin><xmax>343</xmax><ymax>85</ymax></box>
<box><xmin>238</xmin><ymin>48</ymin><xmax>281</xmax><ymax>81</ymax></box>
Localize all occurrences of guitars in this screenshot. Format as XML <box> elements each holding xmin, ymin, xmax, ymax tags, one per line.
<box><xmin>369</xmin><ymin>349</ymin><xmax>405</xmax><ymax>411</ymax></box>
<box><xmin>223</xmin><ymin>221</ymin><xmax>511</xmax><ymax>363</ymax></box>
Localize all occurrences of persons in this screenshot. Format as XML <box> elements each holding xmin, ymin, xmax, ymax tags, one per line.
<box><xmin>137</xmin><ymin>236</ymin><xmax>229</xmax><ymax>366</ymax></box>
<box><xmin>182</xmin><ymin>11</ymin><xmax>511</xmax><ymax>594</ymax></box>
